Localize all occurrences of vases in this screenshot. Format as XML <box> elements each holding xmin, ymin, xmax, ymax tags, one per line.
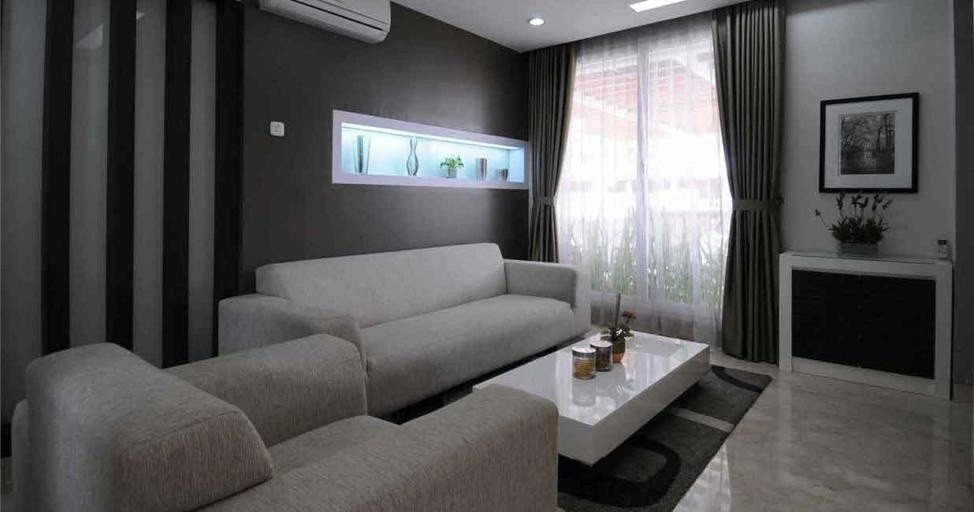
<box><xmin>600</xmin><ymin>336</ymin><xmax>625</xmax><ymax>363</ymax></box>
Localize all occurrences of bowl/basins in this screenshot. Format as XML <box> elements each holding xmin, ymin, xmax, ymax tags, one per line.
<box><xmin>495</xmin><ymin>169</ymin><xmax>508</xmax><ymax>182</ymax></box>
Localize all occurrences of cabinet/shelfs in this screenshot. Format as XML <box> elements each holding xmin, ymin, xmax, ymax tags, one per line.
<box><xmin>331</xmin><ymin>109</ymin><xmax>530</xmax><ymax>190</ymax></box>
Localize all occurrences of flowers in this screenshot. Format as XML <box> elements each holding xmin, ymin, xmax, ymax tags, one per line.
<box><xmin>597</xmin><ymin>292</ymin><xmax>638</xmax><ymax>342</ymax></box>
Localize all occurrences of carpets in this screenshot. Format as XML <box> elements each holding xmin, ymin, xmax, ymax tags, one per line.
<box><xmin>380</xmin><ymin>325</ymin><xmax>773</xmax><ymax>512</ymax></box>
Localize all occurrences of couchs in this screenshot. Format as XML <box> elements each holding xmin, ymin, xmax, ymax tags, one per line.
<box><xmin>218</xmin><ymin>241</ymin><xmax>590</xmax><ymax>416</ymax></box>
<box><xmin>10</xmin><ymin>333</ymin><xmax>559</xmax><ymax>512</ymax></box>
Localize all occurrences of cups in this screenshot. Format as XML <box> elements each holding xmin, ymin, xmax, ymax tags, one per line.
<box><xmin>353</xmin><ymin>135</ymin><xmax>370</xmax><ymax>175</ymax></box>
<box><xmin>476</xmin><ymin>159</ymin><xmax>487</xmax><ymax>180</ymax></box>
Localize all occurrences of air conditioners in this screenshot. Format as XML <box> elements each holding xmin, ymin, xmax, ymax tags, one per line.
<box><xmin>259</xmin><ymin>0</ymin><xmax>392</xmax><ymax>45</ymax></box>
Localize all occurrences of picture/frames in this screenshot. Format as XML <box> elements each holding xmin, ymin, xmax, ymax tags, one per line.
<box><xmin>819</xmin><ymin>91</ymin><xmax>919</xmax><ymax>193</ymax></box>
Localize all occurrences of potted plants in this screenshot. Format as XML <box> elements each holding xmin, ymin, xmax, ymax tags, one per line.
<box><xmin>438</xmin><ymin>151</ymin><xmax>465</xmax><ymax>179</ymax></box>
<box><xmin>815</xmin><ymin>193</ymin><xmax>893</xmax><ymax>262</ymax></box>
